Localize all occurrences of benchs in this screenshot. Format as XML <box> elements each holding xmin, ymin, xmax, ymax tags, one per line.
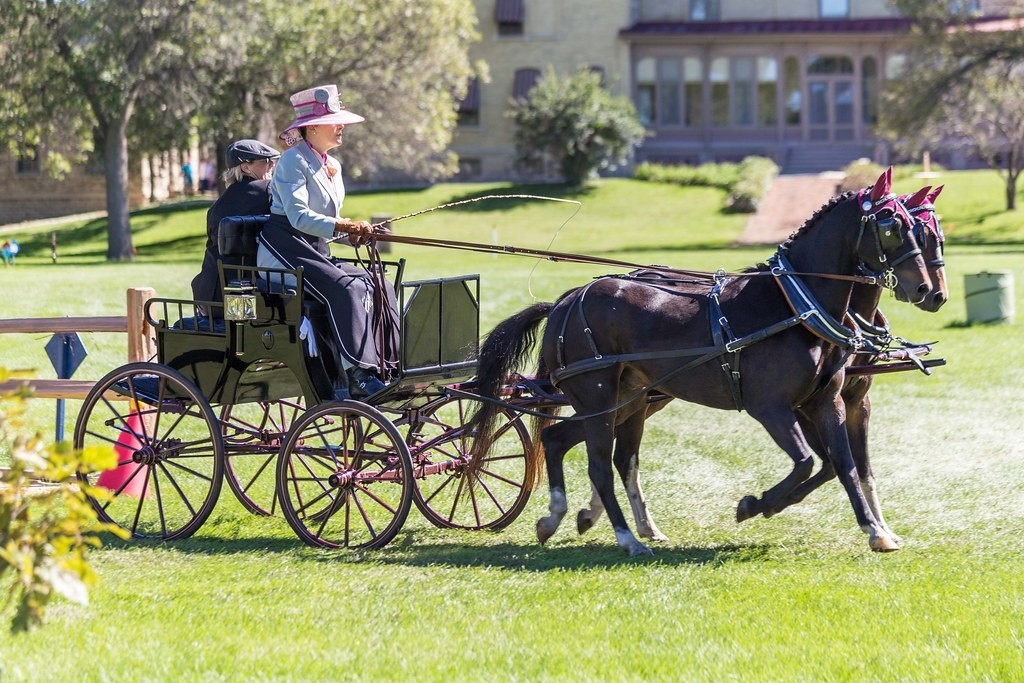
<box><xmin>216</xmin><ymin>213</ymin><xmax>406</xmax><ymax>327</ymax></box>
<box><xmin>144</xmin><ymin>298</ymin><xmax>228</xmax><ymax>338</ymax></box>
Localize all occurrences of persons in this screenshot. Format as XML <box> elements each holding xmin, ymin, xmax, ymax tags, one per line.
<box><xmin>181</xmin><ymin>158</ymin><xmax>194</xmax><ymax>195</ymax></box>
<box><xmin>254</xmin><ymin>85</ymin><xmax>399</xmax><ymax>395</ymax></box>
<box><xmin>191</xmin><ymin>139</ymin><xmax>281</xmax><ymax>319</ymax></box>
<box><xmin>200</xmin><ymin>157</ymin><xmax>216</xmax><ymax>194</ymax></box>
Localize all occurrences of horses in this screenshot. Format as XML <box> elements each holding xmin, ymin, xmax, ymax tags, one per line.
<box><xmin>453</xmin><ymin>165</ymin><xmax>950</xmax><ymax>557</ymax></box>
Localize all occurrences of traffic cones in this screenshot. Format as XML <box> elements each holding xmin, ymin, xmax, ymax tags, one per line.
<box><xmin>93</xmin><ymin>399</ymin><xmax>151</xmax><ymax>500</ymax></box>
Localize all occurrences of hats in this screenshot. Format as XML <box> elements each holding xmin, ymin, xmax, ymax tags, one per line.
<box><xmin>224</xmin><ymin>139</ymin><xmax>281</xmax><ymax>169</ymax></box>
<box><xmin>278</xmin><ymin>84</ymin><xmax>366</xmax><ymax>147</ymax></box>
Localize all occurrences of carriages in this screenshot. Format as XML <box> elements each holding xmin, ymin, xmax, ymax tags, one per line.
<box><xmin>74</xmin><ymin>166</ymin><xmax>948</xmax><ymax>557</ymax></box>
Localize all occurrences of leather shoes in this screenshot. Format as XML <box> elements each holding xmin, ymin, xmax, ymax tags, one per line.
<box><xmin>348</xmin><ymin>366</ymin><xmax>387</xmax><ymax>400</ymax></box>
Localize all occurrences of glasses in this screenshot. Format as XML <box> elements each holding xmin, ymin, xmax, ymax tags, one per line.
<box><xmin>251</xmin><ymin>157</ymin><xmax>271</xmax><ymax>164</ymax></box>
